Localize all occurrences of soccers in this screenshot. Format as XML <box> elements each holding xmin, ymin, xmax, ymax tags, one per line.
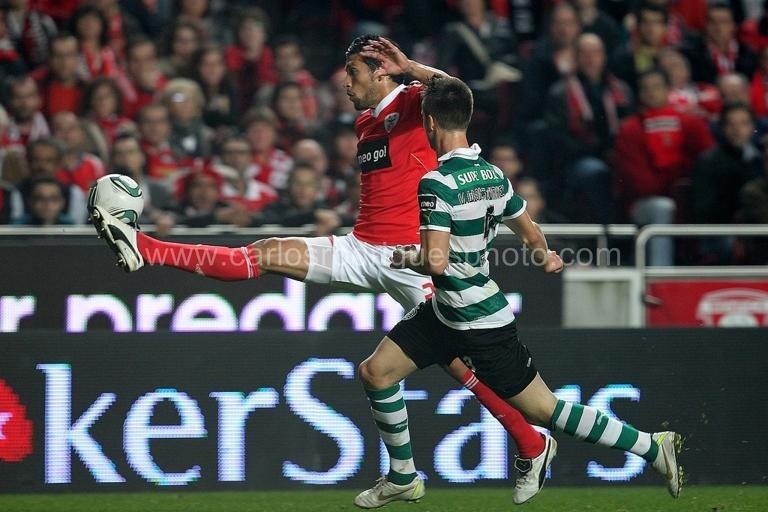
<box><xmin>88</xmin><ymin>175</ymin><xmax>145</xmax><ymax>223</ymax></box>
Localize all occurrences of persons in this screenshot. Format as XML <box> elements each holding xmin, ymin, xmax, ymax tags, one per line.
<box><xmin>352</xmin><ymin>74</ymin><xmax>682</xmax><ymax>511</ymax></box>
<box><xmin>88</xmin><ymin>31</ymin><xmax>558</xmax><ymax>510</ymax></box>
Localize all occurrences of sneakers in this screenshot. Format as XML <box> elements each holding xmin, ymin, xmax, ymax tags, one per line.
<box><xmin>354</xmin><ymin>475</ymin><xmax>425</xmax><ymax>509</ymax></box>
<box><xmin>650</xmin><ymin>431</ymin><xmax>684</xmax><ymax>499</ymax></box>
<box><xmin>513</xmin><ymin>432</ymin><xmax>557</xmax><ymax>504</ymax></box>
<box><xmin>88</xmin><ymin>205</ymin><xmax>145</xmax><ymax>272</ymax></box>
